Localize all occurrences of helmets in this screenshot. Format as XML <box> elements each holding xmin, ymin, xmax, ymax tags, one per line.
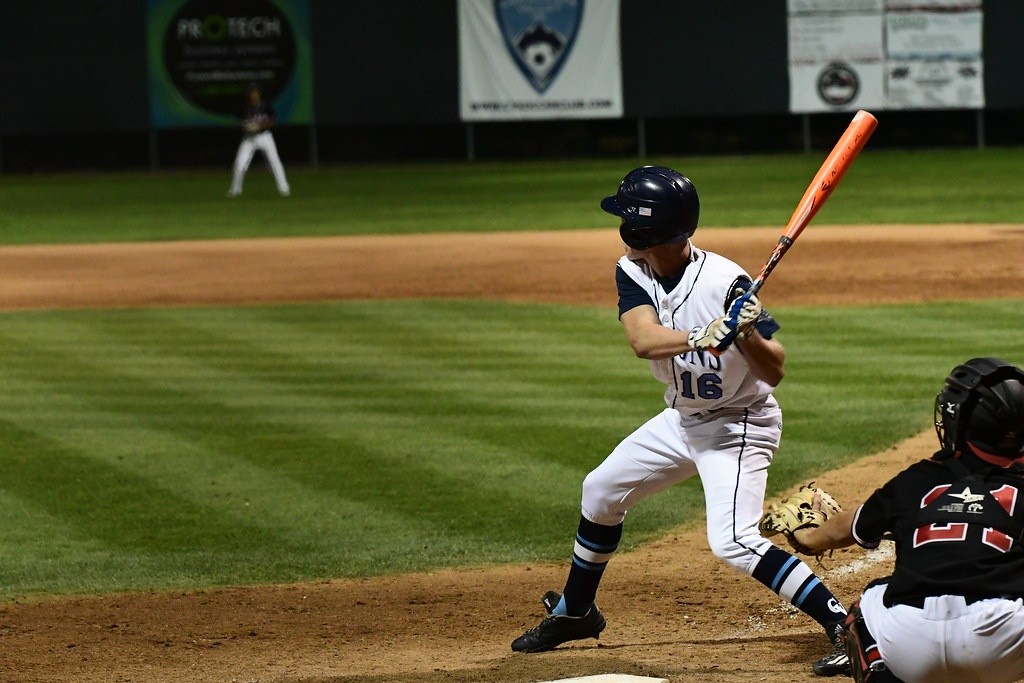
<box><xmin>601</xmin><ymin>167</ymin><xmax>700</xmax><ymax>251</ymax></box>
<box><xmin>934</xmin><ymin>359</ymin><xmax>1024</xmax><ymax>471</ymax></box>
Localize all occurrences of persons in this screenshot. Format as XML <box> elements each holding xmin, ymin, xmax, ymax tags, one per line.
<box><xmin>228</xmin><ymin>81</ymin><xmax>290</xmax><ymax>194</ymax></box>
<box><xmin>756</xmin><ymin>356</ymin><xmax>1024</xmax><ymax>683</ymax></box>
<box><xmin>511</xmin><ymin>166</ymin><xmax>851</xmax><ymax>676</ymax></box>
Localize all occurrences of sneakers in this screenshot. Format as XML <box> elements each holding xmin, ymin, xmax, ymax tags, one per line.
<box><xmin>812</xmin><ymin>624</ymin><xmax>851</xmax><ymax>675</ymax></box>
<box><xmin>510</xmin><ymin>591</ymin><xmax>607</xmax><ymax>653</ymax></box>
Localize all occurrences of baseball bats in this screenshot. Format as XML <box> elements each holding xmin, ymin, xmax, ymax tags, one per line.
<box><xmin>710</xmin><ymin>109</ymin><xmax>878</xmax><ymax>356</ymax></box>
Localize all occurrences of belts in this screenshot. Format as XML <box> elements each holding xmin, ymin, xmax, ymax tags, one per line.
<box><xmin>897</xmin><ymin>593</ymin><xmax>1014</xmax><ymax>608</ymax></box>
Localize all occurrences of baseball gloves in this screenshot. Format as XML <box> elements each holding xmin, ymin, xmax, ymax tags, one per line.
<box><xmin>761</xmin><ymin>481</ymin><xmax>847</xmax><ymax>558</ymax></box>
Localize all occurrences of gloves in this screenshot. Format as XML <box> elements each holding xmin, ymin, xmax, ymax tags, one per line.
<box><xmin>688</xmin><ymin>318</ymin><xmax>746</xmax><ymax>358</ymax></box>
<box><xmin>725</xmin><ymin>288</ymin><xmax>762</xmax><ymax>342</ymax></box>
